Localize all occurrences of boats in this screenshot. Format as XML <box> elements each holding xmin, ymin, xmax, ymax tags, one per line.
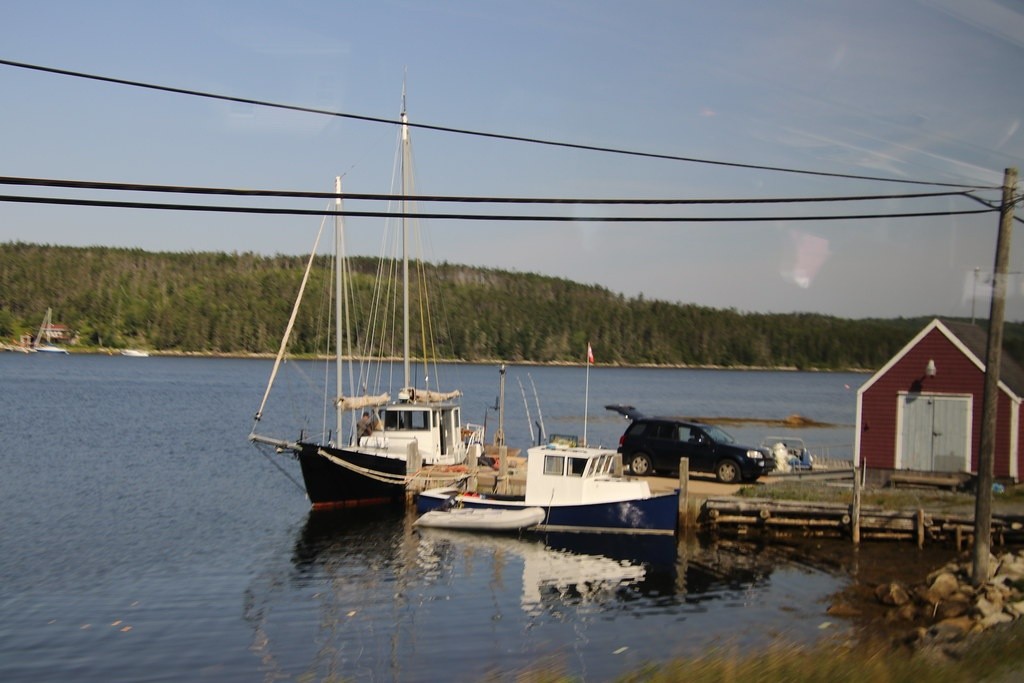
<box><xmin>760</xmin><ymin>438</ymin><xmax>816</xmax><ymax>473</ymax></box>
<box><xmin>121</xmin><ymin>348</ymin><xmax>150</xmax><ymax>360</ymax></box>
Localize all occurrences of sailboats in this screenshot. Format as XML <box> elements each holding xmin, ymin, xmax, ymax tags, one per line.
<box><xmin>1</xmin><ymin>307</ymin><xmax>71</xmax><ymax>356</ymax></box>
<box><xmin>250</xmin><ymin>89</ymin><xmax>679</xmax><ymax>542</ymax></box>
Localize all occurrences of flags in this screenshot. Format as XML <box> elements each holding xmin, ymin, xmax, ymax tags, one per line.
<box><xmin>588</xmin><ymin>345</ymin><xmax>595</xmax><ymax>364</ymax></box>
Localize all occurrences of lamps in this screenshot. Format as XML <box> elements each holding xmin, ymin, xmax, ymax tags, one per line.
<box><xmin>925</xmin><ymin>360</ymin><xmax>937</xmax><ymax>378</ymax></box>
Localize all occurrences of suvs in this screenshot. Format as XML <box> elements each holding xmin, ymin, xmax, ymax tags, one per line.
<box><xmin>602</xmin><ymin>401</ymin><xmax>780</xmax><ymax>484</ymax></box>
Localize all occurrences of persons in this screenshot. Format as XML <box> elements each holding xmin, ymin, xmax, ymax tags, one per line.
<box><xmin>784</xmin><ymin>443</ymin><xmax>813</xmax><ymax>470</ymax></box>
<box><xmin>348</xmin><ymin>411</ymin><xmax>371</xmax><ymax>446</ymax></box>
